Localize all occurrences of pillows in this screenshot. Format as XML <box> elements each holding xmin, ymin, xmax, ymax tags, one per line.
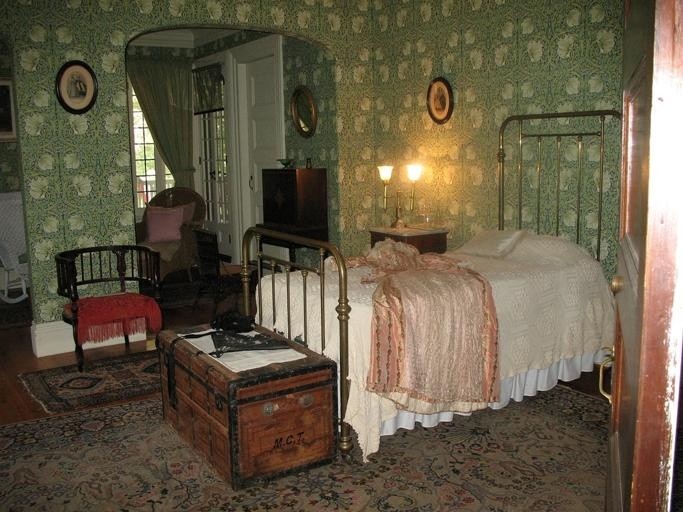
<box><xmin>146</xmin><ymin>202</ymin><xmax>196</xmax><ymax>244</ymax></box>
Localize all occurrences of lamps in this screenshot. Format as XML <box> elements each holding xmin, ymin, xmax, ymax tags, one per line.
<box><xmin>377</xmin><ymin>165</ymin><xmax>422</xmax><ymax>228</ymax></box>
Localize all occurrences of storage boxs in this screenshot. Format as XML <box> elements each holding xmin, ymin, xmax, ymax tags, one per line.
<box><xmin>155</xmin><ymin>323</ymin><xmax>338</xmax><ymax>492</ymax></box>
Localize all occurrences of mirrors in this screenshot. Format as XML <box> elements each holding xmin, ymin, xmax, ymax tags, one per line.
<box><xmin>290</xmin><ymin>85</ymin><xmax>317</xmax><ymax>139</ymax></box>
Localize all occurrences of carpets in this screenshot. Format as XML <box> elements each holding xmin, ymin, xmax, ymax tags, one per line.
<box><xmin>0</xmin><ymin>380</ymin><xmax>611</xmax><ymax>512</ymax></box>
<box><xmin>15</xmin><ymin>350</ymin><xmax>213</xmax><ymax>416</ymax></box>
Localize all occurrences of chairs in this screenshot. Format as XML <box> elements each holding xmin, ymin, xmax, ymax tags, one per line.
<box><xmin>55</xmin><ymin>245</ymin><xmax>165</xmax><ymax>372</ymax></box>
<box><xmin>192</xmin><ymin>228</ymin><xmax>258</xmax><ymax>320</ymax></box>
<box><xmin>136</xmin><ymin>187</ymin><xmax>206</xmax><ymax>291</ymax></box>
<box><xmin>0</xmin><ymin>239</ymin><xmax>29</xmax><ymax>304</ymax></box>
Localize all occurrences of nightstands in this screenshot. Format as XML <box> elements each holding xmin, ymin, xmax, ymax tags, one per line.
<box><xmin>369</xmin><ymin>229</ymin><xmax>449</xmax><ymax>254</ymax></box>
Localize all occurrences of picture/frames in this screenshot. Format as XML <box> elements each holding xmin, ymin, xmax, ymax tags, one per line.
<box><xmin>0</xmin><ymin>77</ymin><xmax>16</xmax><ymax>142</ymax></box>
<box><xmin>55</xmin><ymin>60</ymin><xmax>98</xmax><ymax>115</ymax></box>
<box><xmin>426</xmin><ymin>77</ymin><xmax>454</xmax><ymax>125</ymax></box>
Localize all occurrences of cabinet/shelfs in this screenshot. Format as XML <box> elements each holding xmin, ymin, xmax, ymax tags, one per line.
<box><xmin>256</xmin><ymin>168</ymin><xmax>328</xmax><ymax>277</ymax></box>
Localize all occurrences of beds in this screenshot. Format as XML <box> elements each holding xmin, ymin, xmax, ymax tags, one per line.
<box><xmin>240</xmin><ymin>110</ymin><xmax>622</xmax><ymax>463</ymax></box>
